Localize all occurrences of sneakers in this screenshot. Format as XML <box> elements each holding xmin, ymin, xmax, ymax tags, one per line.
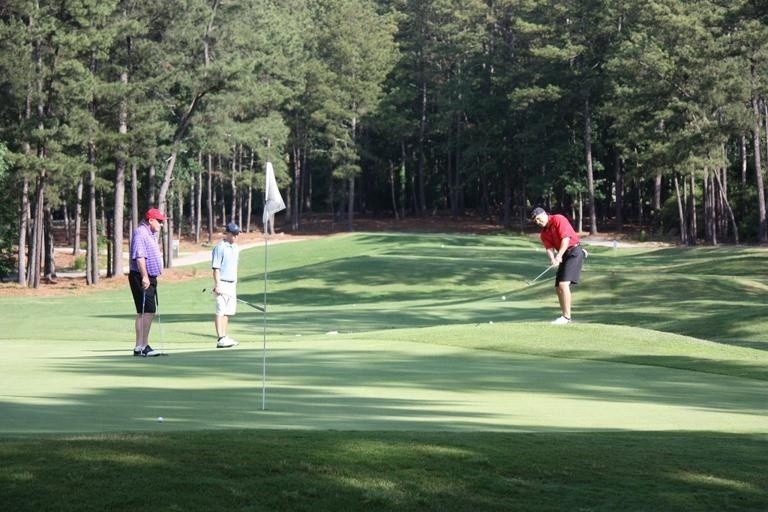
<box><xmin>551</xmin><ymin>316</ymin><xmax>571</xmax><ymax>324</ymax></box>
<box><xmin>217</xmin><ymin>336</ymin><xmax>237</xmax><ymax>347</ymax></box>
<box><xmin>134</xmin><ymin>345</ymin><xmax>160</xmax><ymax>356</ymax></box>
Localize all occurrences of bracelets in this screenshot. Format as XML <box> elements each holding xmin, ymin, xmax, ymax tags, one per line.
<box><xmin>556</xmin><ymin>256</ymin><xmax>563</xmax><ymax>262</ymax></box>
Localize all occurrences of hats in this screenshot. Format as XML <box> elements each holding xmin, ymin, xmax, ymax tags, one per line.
<box><xmin>145</xmin><ymin>208</ymin><xmax>168</xmax><ymax>221</ymax></box>
<box><xmin>531</xmin><ymin>207</ymin><xmax>544</xmax><ymax>219</ymax></box>
<box><xmin>227</xmin><ymin>223</ymin><xmax>240</xmax><ymax>235</ymax></box>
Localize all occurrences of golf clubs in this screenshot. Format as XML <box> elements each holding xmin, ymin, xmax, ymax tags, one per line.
<box><xmin>524</xmin><ymin>265</ymin><xmax>554</xmax><ymax>285</ymax></box>
<box><xmin>138</xmin><ymin>288</ymin><xmax>146</xmax><ymax>357</ymax></box>
<box><xmin>156</xmin><ymin>289</ymin><xmax>167</xmax><ymax>355</ymax></box>
<box><xmin>202</xmin><ymin>288</ymin><xmax>264</xmax><ymax>311</ymax></box>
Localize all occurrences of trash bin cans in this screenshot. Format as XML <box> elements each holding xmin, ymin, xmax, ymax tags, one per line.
<box><xmin>173</xmin><ymin>239</ymin><xmax>179</xmax><ymax>257</ymax></box>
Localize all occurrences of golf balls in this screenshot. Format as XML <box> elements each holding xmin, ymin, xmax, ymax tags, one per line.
<box><xmin>501</xmin><ymin>295</ymin><xmax>506</xmax><ymax>301</ymax></box>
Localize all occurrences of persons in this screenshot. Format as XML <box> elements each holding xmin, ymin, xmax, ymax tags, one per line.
<box><xmin>531</xmin><ymin>207</ymin><xmax>584</xmax><ymax>325</ymax></box>
<box><xmin>212</xmin><ymin>223</ymin><xmax>242</xmax><ymax>348</ymax></box>
<box><xmin>128</xmin><ymin>209</ymin><xmax>169</xmax><ymax>356</ymax></box>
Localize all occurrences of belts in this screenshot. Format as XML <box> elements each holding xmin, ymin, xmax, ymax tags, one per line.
<box><xmin>221</xmin><ymin>280</ymin><xmax>234</xmax><ymax>283</ymax></box>
